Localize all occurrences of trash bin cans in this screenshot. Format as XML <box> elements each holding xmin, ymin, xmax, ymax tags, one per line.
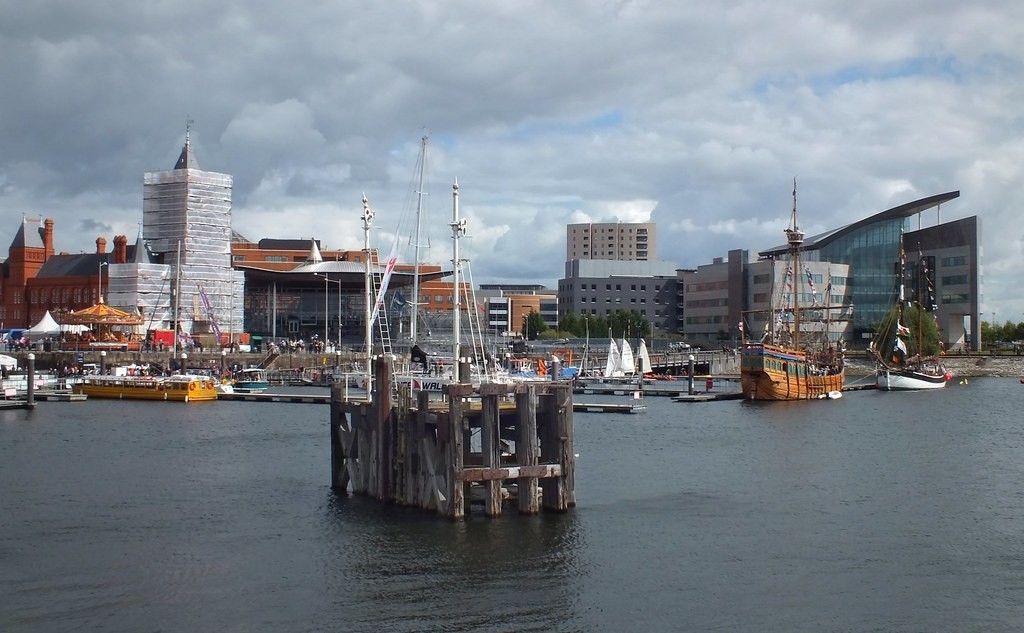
<box><xmin>705</xmin><ymin>376</ymin><xmax>715</xmax><ymax>391</ymax></box>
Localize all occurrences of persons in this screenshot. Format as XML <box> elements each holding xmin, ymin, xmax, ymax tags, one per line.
<box><xmin>55</xmin><ymin>356</ymin><xmax>68</xmax><ymax>378</ymax></box>
<box><xmin>8</xmin><ymin>336</ymin><xmax>14</xmax><ymax>351</ymax></box>
<box><xmin>268</xmin><ymin>336</ymin><xmax>338</xmax><ymax>353</ymax></box>
<box><xmin>25</xmin><ymin>337</ymin><xmax>31</xmax><ymax>350</ymax></box>
<box><xmin>12</xmin><ymin>365</ymin><xmax>15</xmax><ymax>371</ymax></box>
<box><xmin>233</xmin><ymin>340</ymin><xmax>240</xmax><ymax>355</ymax></box>
<box><xmin>18</xmin><ymin>366</ymin><xmax>21</xmax><ymax>371</ymax></box>
<box><xmin>438</xmin><ymin>360</ymin><xmax>444</xmax><ymax>372</ymax></box>
<box><xmin>159</xmin><ymin>338</ymin><xmax>164</xmax><ymax>352</ymax></box>
<box><xmin>159</xmin><ymin>383</ymin><xmax>162</xmax><ymax>390</ymax></box>
<box><xmin>130</xmin><ymin>367</ymin><xmax>146</xmax><ymax>375</ymax></box>
<box><xmin>14</xmin><ymin>338</ymin><xmax>20</xmax><ymax>350</ymax></box>
<box><xmin>151</xmin><ymin>383</ymin><xmax>154</xmax><ymax>389</ymax></box>
<box><xmin>807</xmin><ymin>365</ymin><xmax>838</xmax><ymax>376</ymax></box>
<box><xmin>194</xmin><ymin>337</ymin><xmax>203</xmax><ymax>352</ymax></box>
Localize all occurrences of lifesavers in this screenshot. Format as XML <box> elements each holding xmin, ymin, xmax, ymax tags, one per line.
<box><xmin>207</xmin><ymin>382</ymin><xmax>214</xmax><ymax>389</ymax></box>
<box><xmin>190</xmin><ymin>384</ymin><xmax>196</xmax><ymax>391</ymax></box>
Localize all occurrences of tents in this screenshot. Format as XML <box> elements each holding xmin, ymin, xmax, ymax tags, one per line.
<box><xmin>23</xmin><ymin>310</ymin><xmax>60</xmax><ymax>341</ymax></box>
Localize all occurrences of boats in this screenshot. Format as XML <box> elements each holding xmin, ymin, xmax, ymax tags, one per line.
<box><xmin>19</xmin><ymin>391</ymin><xmax>89</xmax><ymax>402</ymax></box>
<box><xmin>231</xmin><ymin>365</ymin><xmax>269</xmax><ymax>392</ymax></box>
<box><xmin>70</xmin><ymin>373</ymin><xmax>219</xmax><ymax>402</ymax></box>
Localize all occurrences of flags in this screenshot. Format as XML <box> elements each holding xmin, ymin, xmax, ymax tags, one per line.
<box><xmin>892</xmin><ymin>228</ymin><xmax>947</xmax><ymax>374</ymax></box>
<box><xmin>391</xmin><ymin>291</ymin><xmax>406</xmax><ymax>313</ymax></box>
<box><xmin>737</xmin><ymin>257</ymin><xmax>830</xmax><ymax>349</ymax></box>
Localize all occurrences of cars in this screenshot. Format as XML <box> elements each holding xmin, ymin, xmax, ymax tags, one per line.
<box><xmin>673</xmin><ymin>342</ymin><xmax>691</xmax><ymax>349</ymax></box>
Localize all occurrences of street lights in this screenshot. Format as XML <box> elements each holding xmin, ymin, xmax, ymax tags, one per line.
<box><xmin>28</xmin><ymin>325</ymin><xmax>31</xmax><ymax>350</ymax></box>
<box><xmin>100</xmin><ymin>351</ymin><xmax>107</xmax><ymax>375</ymax></box>
<box><xmin>325</xmin><ymin>278</ymin><xmax>342</xmax><ymax>351</ymax></box>
<box><xmin>314</xmin><ymin>272</ymin><xmax>328</xmax><ymax>350</ymax></box>
<box><xmin>59</xmin><ymin>305</ymin><xmax>64</xmax><ymax>350</ymax></box>
<box><xmin>688</xmin><ymin>354</ymin><xmax>695</xmax><ymax>395</ymax></box>
<box><xmin>221</xmin><ymin>350</ymin><xmax>227</xmax><ymax>369</ymax></box>
<box><xmin>27</xmin><ymin>351</ymin><xmax>35</xmax><ymax>404</ymax></box>
<box><xmin>585</xmin><ymin>317</ymin><xmax>589</xmax><ymax>346</ymax></box>
<box><xmin>992</xmin><ymin>312</ymin><xmax>996</xmax><ymax>327</ymax></box>
<box><xmin>99</xmin><ymin>261</ymin><xmax>107</xmax><ymax>303</ymax></box>
<box><xmin>522</xmin><ymin>313</ymin><xmax>529</xmax><ymax>342</ymax></box>
<box><xmin>609</xmin><ymin>327</ymin><xmax>611</xmax><ymax>345</ymax></box>
<box><xmin>180</xmin><ymin>352</ymin><xmax>188</xmax><ymax>374</ymax></box>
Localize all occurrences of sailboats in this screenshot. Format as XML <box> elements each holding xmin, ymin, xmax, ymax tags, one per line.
<box><xmin>866</xmin><ymin>227</ymin><xmax>951</xmax><ymax>390</ymax></box>
<box><xmin>130</xmin><ymin>238</ymin><xmax>232</xmax><ymax>392</ymax></box>
<box><xmin>740</xmin><ymin>175</ymin><xmax>844</xmax><ymax>401</ymax></box>
<box><xmin>352</xmin><ymin>123</ymin><xmax>652</xmax><ymax>390</ymax></box>
